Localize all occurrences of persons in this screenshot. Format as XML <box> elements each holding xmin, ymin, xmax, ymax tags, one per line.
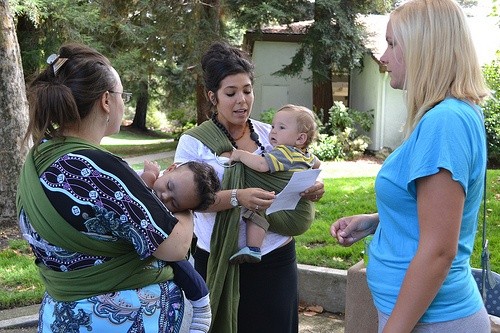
<box><xmin>141</xmin><ymin>159</ymin><xmax>220</xmax><ymax>333</ymax></box>
<box><xmin>330</xmin><ymin>0</ymin><xmax>490</xmax><ymax>333</ymax></box>
<box><xmin>173</xmin><ymin>43</ymin><xmax>324</xmax><ymax>333</ymax></box>
<box><xmin>16</xmin><ymin>43</ymin><xmax>194</xmax><ymax>333</ymax></box>
<box><xmin>229</xmin><ymin>104</ymin><xmax>315</xmax><ymax>263</ymax></box>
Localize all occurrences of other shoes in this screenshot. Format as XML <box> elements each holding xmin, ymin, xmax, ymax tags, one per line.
<box><xmin>230</xmin><ymin>247</ymin><xmax>262</xmax><ymax>264</ymax></box>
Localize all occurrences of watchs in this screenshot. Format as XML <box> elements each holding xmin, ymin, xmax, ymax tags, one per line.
<box><xmin>230</xmin><ymin>189</ymin><xmax>239</xmax><ymax>207</ymax></box>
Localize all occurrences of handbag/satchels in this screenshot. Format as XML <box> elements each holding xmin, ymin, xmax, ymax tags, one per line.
<box><xmin>472</xmin><ymin>267</ymin><xmax>500</xmax><ymax>317</ymax></box>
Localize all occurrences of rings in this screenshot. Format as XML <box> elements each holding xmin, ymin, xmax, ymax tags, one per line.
<box><xmin>316</xmin><ymin>195</ymin><xmax>317</xmax><ymax>199</ymax></box>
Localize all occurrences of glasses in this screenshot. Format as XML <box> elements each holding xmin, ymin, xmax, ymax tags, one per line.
<box><xmin>107</xmin><ymin>89</ymin><xmax>133</xmax><ymax>104</ymax></box>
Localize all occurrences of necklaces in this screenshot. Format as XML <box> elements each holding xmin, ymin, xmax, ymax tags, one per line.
<box><xmin>211</xmin><ymin>111</ymin><xmax>265</xmax><ymax>156</ymax></box>
